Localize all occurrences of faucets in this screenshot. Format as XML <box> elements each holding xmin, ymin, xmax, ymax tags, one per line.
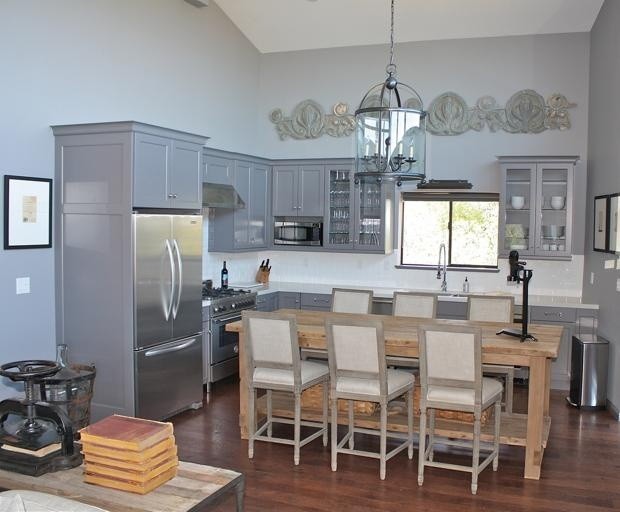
<box><xmin>436</xmin><ymin>244</ymin><xmax>447</xmax><ymax>290</ymax></box>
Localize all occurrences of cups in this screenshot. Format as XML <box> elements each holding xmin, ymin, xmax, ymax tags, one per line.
<box><xmin>512</xmin><ymin>196</ymin><xmax>525</xmax><ymax>209</ymax></box>
<box><xmin>542</xmin><ymin>244</ymin><xmax>564</xmax><ymax>250</ymax></box>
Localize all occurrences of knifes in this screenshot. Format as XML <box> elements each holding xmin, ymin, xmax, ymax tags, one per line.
<box><xmin>260</xmin><ymin>258</ymin><xmax>271</xmax><ymax>272</ymax></box>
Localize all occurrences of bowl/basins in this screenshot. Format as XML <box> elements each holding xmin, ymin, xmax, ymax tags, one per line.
<box><xmin>551</xmin><ymin>196</ymin><xmax>565</xmax><ymax>209</ymax></box>
<box><xmin>542</xmin><ymin>225</ymin><xmax>564</xmax><ymax>237</ymax></box>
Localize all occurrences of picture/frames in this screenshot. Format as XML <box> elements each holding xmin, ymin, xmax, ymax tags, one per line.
<box><xmin>3</xmin><ymin>175</ymin><xmax>53</xmax><ymax>250</ymax></box>
<box><xmin>593</xmin><ymin>193</ymin><xmax>620</xmax><ymax>254</ymax></box>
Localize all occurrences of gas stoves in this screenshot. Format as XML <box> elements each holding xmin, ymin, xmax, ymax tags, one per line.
<box><xmin>202</xmin><ymin>286</ymin><xmax>257</xmax><ymax>317</ymax></box>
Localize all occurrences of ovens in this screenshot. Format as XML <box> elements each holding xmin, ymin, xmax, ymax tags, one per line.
<box><xmin>210</xmin><ymin>312</ymin><xmax>242</xmax><ymax>364</ymax></box>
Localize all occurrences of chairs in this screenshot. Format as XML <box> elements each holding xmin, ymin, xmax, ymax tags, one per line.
<box><xmin>467</xmin><ymin>295</ymin><xmax>515</xmax><ymax>413</ymax></box>
<box><xmin>240</xmin><ymin>309</ymin><xmax>329</xmax><ymax>465</ymax></box>
<box><xmin>389</xmin><ymin>291</ymin><xmax>438</xmax><ymax>370</ymax></box>
<box><xmin>299</xmin><ymin>288</ymin><xmax>373</xmax><ymax>361</ymax></box>
<box><xmin>324</xmin><ymin>318</ymin><xmax>416</xmax><ymax>480</ymax></box>
<box><xmin>416</xmin><ymin>324</ymin><xmax>504</xmax><ymax>495</ymax></box>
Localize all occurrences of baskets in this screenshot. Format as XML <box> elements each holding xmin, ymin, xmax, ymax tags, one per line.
<box><xmin>28</xmin><ymin>360</ymin><xmax>99</xmax><ymax>431</ymax></box>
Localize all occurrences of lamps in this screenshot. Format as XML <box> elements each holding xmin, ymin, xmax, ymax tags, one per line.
<box><xmin>353</xmin><ymin>1</ymin><xmax>427</xmax><ymax>186</ymax></box>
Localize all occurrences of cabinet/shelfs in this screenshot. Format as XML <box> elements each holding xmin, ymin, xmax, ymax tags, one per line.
<box><xmin>279</xmin><ymin>292</ymin><xmax>301</xmax><ymax>309</ymax></box>
<box><xmin>204</xmin><ymin>147</ymin><xmax>234</xmax><ymax>186</ymax></box>
<box><xmin>49</xmin><ymin>121</ymin><xmax>211</xmax><ymax>210</ymax></box>
<box><xmin>437</xmin><ymin>301</ymin><xmax>468</xmax><ymax>320</ymax></box>
<box><xmin>530</xmin><ymin>307</ymin><xmax>575</xmax><ymax>391</ymax></box>
<box><xmin>257</xmin><ymin>293</ymin><xmax>278</xmax><ymax>312</ymax></box>
<box><xmin>270</xmin><ymin>159</ymin><xmax>324</xmax><ymax>217</ymax></box>
<box><xmin>497</xmin><ymin>156</ymin><xmax>580</xmax><ymax>261</ymax></box>
<box><xmin>324</xmin><ymin>158</ymin><xmax>396</xmax><ymax>254</ymax></box>
<box><xmin>300</xmin><ymin>293</ymin><xmax>332</xmax><ymax>312</ymax></box>
<box><xmin>212</xmin><ymin>152</ymin><xmax>269</xmax><ymax>251</ymax></box>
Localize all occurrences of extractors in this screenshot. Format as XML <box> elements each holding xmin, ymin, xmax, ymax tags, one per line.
<box><xmin>202</xmin><ymin>182</ymin><xmax>245</xmax><ymax>208</ymax></box>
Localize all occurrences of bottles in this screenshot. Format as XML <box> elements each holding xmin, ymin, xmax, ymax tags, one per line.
<box><xmin>221</xmin><ymin>261</ymin><xmax>228</xmax><ymax>288</ymax></box>
<box><xmin>462</xmin><ymin>277</ymin><xmax>469</xmax><ymax>293</ymax></box>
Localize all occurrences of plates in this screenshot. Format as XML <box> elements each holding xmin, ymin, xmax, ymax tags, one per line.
<box><xmin>510</xmin><ymin>244</ymin><xmax>527</xmax><ymax>249</ymax></box>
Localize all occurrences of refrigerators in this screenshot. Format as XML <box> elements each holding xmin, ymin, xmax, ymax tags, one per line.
<box><xmin>132</xmin><ymin>214</ymin><xmax>204</xmax><ymax>421</ymax></box>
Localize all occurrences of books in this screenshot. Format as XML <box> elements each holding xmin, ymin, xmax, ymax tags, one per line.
<box><xmin>76</xmin><ymin>414</ymin><xmax>179</xmax><ymax>495</ymax></box>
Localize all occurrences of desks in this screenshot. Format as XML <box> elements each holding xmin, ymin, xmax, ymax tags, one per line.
<box><xmin>0</xmin><ymin>432</ymin><xmax>245</xmax><ymax>512</ymax></box>
<box><xmin>225</xmin><ymin>308</ymin><xmax>564</xmax><ymax>480</ymax></box>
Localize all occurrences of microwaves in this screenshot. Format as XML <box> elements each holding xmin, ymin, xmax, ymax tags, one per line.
<box><xmin>273</xmin><ymin>216</ymin><xmax>323</xmax><ymax>246</ymax></box>
